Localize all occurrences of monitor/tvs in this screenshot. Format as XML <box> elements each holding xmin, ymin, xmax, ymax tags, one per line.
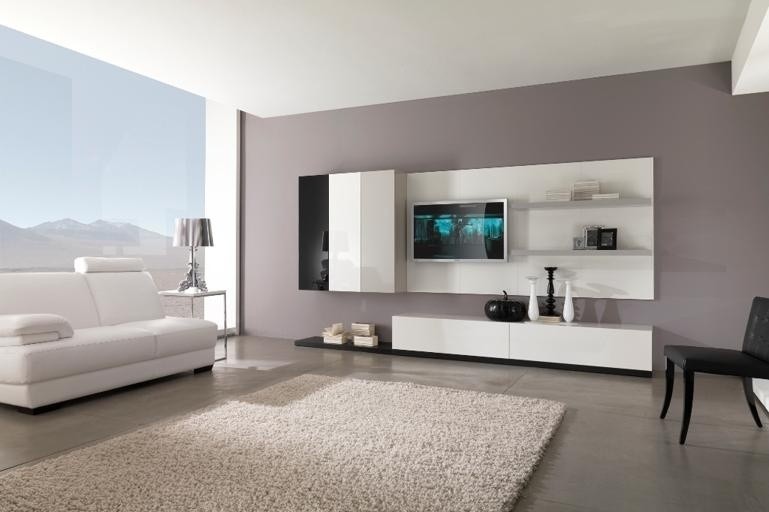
<box><xmin>410</xmin><ymin>199</ymin><xmax>507</xmax><ymax>263</ymax></box>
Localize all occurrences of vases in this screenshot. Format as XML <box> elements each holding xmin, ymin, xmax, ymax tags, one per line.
<box><xmin>563</xmin><ymin>281</ymin><xmax>575</xmax><ymax>322</ymax></box>
<box><xmin>527</xmin><ymin>276</ymin><xmax>540</xmax><ymax>321</ymax></box>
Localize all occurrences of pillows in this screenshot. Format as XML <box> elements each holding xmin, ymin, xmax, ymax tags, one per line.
<box><xmin>73</xmin><ymin>256</ymin><xmax>146</xmax><ymax>273</ymax></box>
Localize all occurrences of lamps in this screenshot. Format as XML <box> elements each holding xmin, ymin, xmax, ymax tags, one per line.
<box><xmin>320</xmin><ymin>231</ymin><xmax>328</xmax><ymax>281</ymax></box>
<box><xmin>171</xmin><ymin>217</ymin><xmax>214</xmax><ymax>292</ymax></box>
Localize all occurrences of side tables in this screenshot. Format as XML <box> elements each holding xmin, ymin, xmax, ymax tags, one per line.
<box><xmin>158</xmin><ymin>288</ymin><xmax>228</xmax><ymax>362</ymax></box>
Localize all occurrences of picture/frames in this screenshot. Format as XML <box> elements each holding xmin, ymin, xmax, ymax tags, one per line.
<box><xmin>583</xmin><ymin>225</ymin><xmax>606</xmax><ymax>249</ymax></box>
<box><xmin>596</xmin><ymin>228</ymin><xmax>617</xmax><ymax>250</ymax></box>
<box><xmin>572</xmin><ymin>236</ymin><xmax>583</xmax><ymax>250</ymax></box>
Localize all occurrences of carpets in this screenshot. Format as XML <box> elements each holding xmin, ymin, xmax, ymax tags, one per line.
<box><xmin>1</xmin><ymin>372</ymin><xmax>568</xmax><ymax>512</ymax></box>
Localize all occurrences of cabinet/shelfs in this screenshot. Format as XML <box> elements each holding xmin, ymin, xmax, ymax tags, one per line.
<box><xmin>510</xmin><ymin>196</ymin><xmax>652</xmax><ymax>257</ymax></box>
<box><xmin>391</xmin><ymin>310</ymin><xmax>655</xmax><ymax>378</ymax></box>
<box><xmin>328</xmin><ymin>169</ymin><xmax>407</xmax><ymax>294</ymax></box>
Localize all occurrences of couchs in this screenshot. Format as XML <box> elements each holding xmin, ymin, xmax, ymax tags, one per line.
<box><xmin>0</xmin><ymin>271</ymin><xmax>217</xmax><ymax>415</ymax></box>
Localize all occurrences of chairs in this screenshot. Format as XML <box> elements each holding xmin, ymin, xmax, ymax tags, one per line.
<box><xmin>659</xmin><ymin>296</ymin><xmax>769</xmax><ymax>444</ymax></box>
